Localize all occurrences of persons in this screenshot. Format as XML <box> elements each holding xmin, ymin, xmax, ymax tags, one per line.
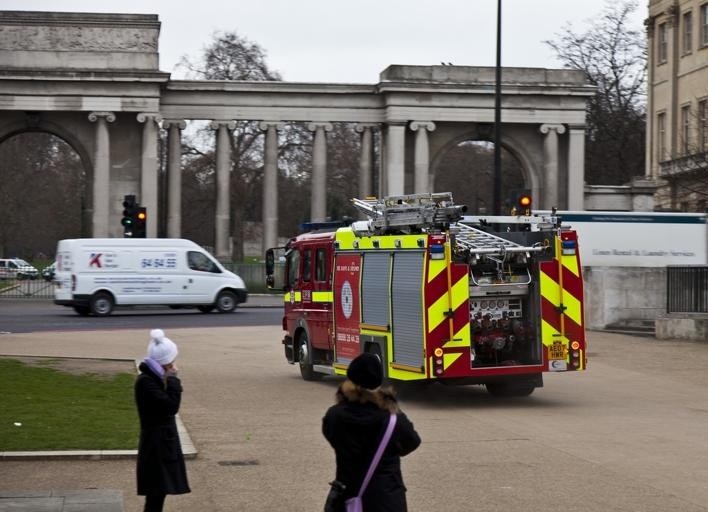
<box><xmin>322</xmin><ymin>351</ymin><xmax>421</xmax><ymax>511</ymax></box>
<box><xmin>135</xmin><ymin>329</ymin><xmax>192</xmax><ymax>512</ymax></box>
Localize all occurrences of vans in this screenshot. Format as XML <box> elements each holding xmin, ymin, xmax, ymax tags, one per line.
<box><xmin>52</xmin><ymin>238</ymin><xmax>248</xmax><ymax>316</ymax></box>
<box><xmin>0</xmin><ymin>259</ymin><xmax>39</xmax><ymax>280</ymax></box>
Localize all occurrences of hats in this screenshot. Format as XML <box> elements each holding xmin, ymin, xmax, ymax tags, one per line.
<box><xmin>347</xmin><ymin>353</ymin><xmax>386</xmax><ymax>388</ymax></box>
<box><xmin>149</xmin><ymin>329</ymin><xmax>179</xmax><ymax>365</ymax></box>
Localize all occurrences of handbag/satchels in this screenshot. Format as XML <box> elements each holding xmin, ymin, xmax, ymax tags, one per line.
<box><xmin>346</xmin><ymin>497</ymin><xmax>362</xmax><ymax>512</ymax></box>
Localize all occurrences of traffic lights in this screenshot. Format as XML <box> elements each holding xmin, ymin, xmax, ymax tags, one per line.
<box><xmin>121</xmin><ymin>197</ymin><xmax>146</xmax><ymax>237</ymax></box>
<box><xmin>517</xmin><ymin>195</ymin><xmax>531</xmax><ymax>217</ymax></box>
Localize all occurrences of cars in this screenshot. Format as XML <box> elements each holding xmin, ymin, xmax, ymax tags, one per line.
<box><xmin>42</xmin><ymin>264</ymin><xmax>56</xmax><ymax>280</ymax></box>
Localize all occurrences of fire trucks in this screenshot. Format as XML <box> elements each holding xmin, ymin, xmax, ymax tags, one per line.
<box><xmin>265</xmin><ymin>191</ymin><xmax>587</xmax><ymax>396</ymax></box>
